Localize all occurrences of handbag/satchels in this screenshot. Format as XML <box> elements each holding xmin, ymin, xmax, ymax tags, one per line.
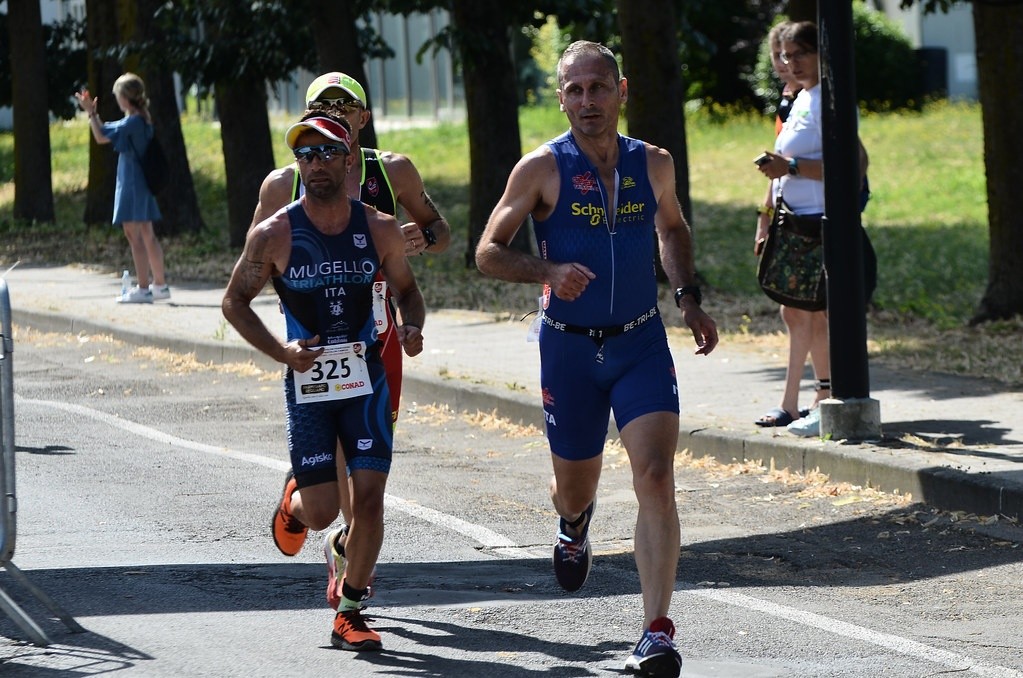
<box><xmin>757</xmin><ymin>229</ymin><xmax>828</xmax><ymax>313</ymax></box>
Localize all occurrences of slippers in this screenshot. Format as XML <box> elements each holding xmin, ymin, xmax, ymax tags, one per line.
<box><xmin>755</xmin><ymin>408</ymin><xmax>794</xmax><ymax>427</ymax></box>
<box><xmin>797</xmin><ymin>408</ymin><xmax>811</xmax><ymax>416</ymax></box>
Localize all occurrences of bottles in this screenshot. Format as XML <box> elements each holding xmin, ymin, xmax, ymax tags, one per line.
<box><xmin>122</xmin><ymin>270</ymin><xmax>131</xmax><ymax>295</ymax></box>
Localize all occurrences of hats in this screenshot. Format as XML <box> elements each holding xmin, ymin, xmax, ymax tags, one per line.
<box><xmin>285</xmin><ymin>117</ymin><xmax>352</xmax><ymax>152</ymax></box>
<box><xmin>305</xmin><ymin>71</ymin><xmax>366</xmax><ymax>109</ymax></box>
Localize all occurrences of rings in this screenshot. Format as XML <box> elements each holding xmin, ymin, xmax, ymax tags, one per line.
<box><xmin>412</xmin><ymin>240</ymin><xmax>415</xmax><ymax>247</ymax></box>
<box><xmin>305</xmin><ymin>340</ymin><xmax>307</xmax><ymax>345</ymax></box>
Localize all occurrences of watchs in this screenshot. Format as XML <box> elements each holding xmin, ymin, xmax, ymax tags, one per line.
<box><xmin>789</xmin><ymin>159</ymin><xmax>799</xmax><ymax>176</ymax></box>
<box><xmin>421</xmin><ymin>227</ymin><xmax>436</xmax><ymax>249</ymax></box>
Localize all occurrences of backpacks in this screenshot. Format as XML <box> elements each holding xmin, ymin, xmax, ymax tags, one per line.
<box><xmin>129</xmin><ymin>116</ymin><xmax>171</xmax><ymax>195</ymax></box>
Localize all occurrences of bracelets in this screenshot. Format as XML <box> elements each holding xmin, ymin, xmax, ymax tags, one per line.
<box><xmin>675</xmin><ymin>286</ymin><xmax>701</xmax><ymax>308</ymax></box>
<box><xmin>402</xmin><ymin>323</ymin><xmax>420</xmax><ymax>328</ymax></box>
<box><xmin>756</xmin><ymin>205</ymin><xmax>772</xmax><ymax>216</ymax></box>
<box><xmin>89</xmin><ymin>113</ymin><xmax>95</xmax><ymax>118</ymax></box>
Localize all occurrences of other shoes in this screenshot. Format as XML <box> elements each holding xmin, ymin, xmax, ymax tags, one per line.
<box><xmin>789</xmin><ymin>405</ymin><xmax>823</xmax><ymax>437</ymax></box>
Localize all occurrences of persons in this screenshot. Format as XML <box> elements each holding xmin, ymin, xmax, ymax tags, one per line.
<box><xmin>246</xmin><ymin>72</ymin><xmax>451</xmax><ymax>612</ymax></box>
<box><xmin>476</xmin><ymin>39</ymin><xmax>721</xmax><ymax>678</ymax></box>
<box><xmin>220</xmin><ymin>112</ymin><xmax>424</xmax><ymax>655</ymax></box>
<box><xmin>76</xmin><ymin>73</ymin><xmax>171</xmax><ymax>304</ymax></box>
<box><xmin>755</xmin><ymin>19</ymin><xmax>870</xmax><ymax>426</ymax></box>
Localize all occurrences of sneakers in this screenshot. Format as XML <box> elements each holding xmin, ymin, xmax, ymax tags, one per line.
<box><xmin>625</xmin><ymin>616</ymin><xmax>682</xmax><ymax>678</ymax></box>
<box><xmin>331</xmin><ymin>605</ymin><xmax>383</xmax><ymax>651</ymax></box>
<box><xmin>149</xmin><ymin>284</ymin><xmax>170</xmax><ymax>299</ymax></box>
<box><xmin>115</xmin><ymin>284</ymin><xmax>154</xmax><ymax>304</ymax></box>
<box><xmin>324</xmin><ymin>524</ymin><xmax>377</xmax><ymax>611</ymax></box>
<box><xmin>552</xmin><ymin>493</ymin><xmax>598</xmax><ymax>591</ymax></box>
<box><xmin>271</xmin><ymin>467</ymin><xmax>309</xmax><ymax>557</ymax></box>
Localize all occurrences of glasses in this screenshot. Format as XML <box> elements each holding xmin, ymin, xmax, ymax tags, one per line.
<box><xmin>780</xmin><ymin>49</ymin><xmax>811</xmax><ymax>64</ymax></box>
<box><xmin>310</xmin><ymin>97</ymin><xmax>365</xmax><ymax>114</ymax></box>
<box><xmin>293</xmin><ymin>144</ymin><xmax>349</xmax><ymax>163</ymax></box>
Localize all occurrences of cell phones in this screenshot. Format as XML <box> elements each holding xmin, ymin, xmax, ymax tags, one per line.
<box><xmin>753</xmin><ymin>154</ymin><xmax>771</xmax><ymax>167</ymax></box>
